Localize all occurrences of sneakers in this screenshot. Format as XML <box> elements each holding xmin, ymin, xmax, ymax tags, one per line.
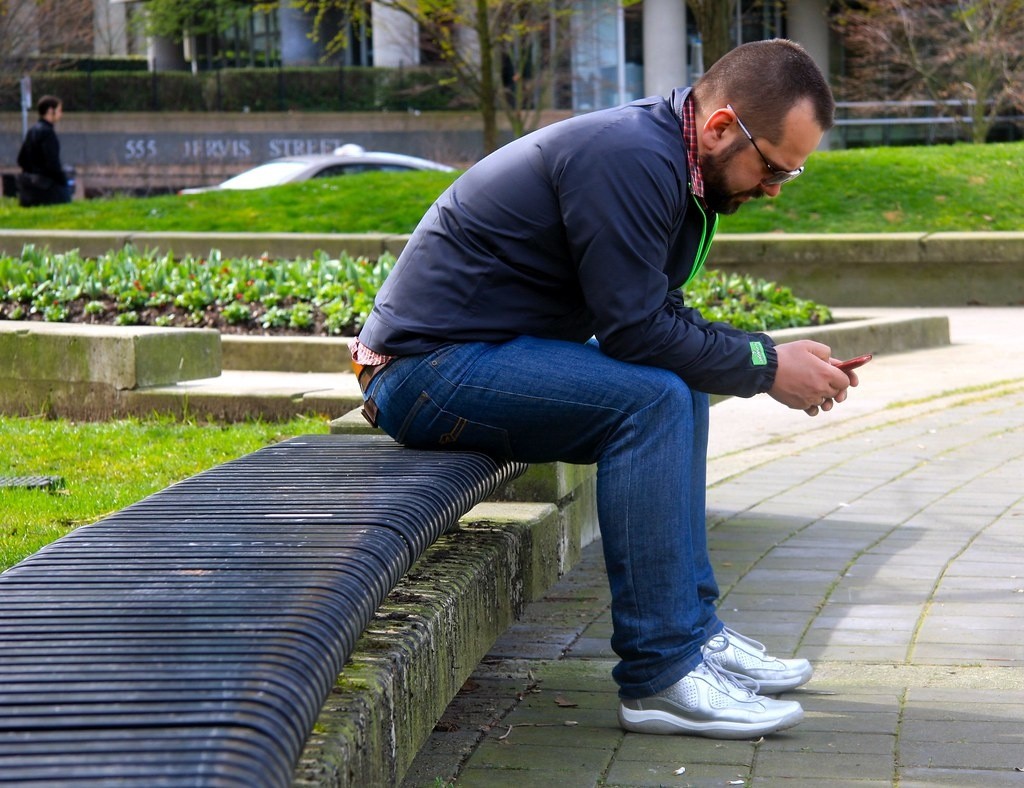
<box><xmin>619</xmin><ymin>662</ymin><xmax>803</xmax><ymax>740</ymax></box>
<box><xmin>701</xmin><ymin>627</ymin><xmax>814</xmax><ymax>695</ymax></box>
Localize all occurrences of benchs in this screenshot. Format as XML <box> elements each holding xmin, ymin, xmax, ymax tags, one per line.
<box><xmin>0</xmin><ymin>431</ymin><xmax>530</xmax><ymax>788</ymax></box>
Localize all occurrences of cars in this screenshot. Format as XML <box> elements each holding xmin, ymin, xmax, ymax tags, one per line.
<box><xmin>177</xmin><ymin>142</ymin><xmax>463</xmax><ymax>197</ymax></box>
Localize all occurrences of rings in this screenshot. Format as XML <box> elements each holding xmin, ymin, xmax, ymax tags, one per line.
<box><xmin>818</xmin><ymin>396</ymin><xmax>826</xmax><ymax>406</ymax></box>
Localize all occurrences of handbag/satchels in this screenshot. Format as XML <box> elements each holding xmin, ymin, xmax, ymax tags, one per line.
<box><xmin>18</xmin><ymin>164</ymin><xmax>53</xmax><ymax>208</ymax></box>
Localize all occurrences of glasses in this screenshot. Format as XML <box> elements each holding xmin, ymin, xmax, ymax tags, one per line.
<box><xmin>728</xmin><ymin>105</ymin><xmax>804</xmax><ymax>186</ymax></box>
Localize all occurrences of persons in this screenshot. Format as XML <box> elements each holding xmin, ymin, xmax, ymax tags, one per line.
<box><xmin>351</xmin><ymin>36</ymin><xmax>858</xmax><ymax>742</ymax></box>
<box><xmin>15</xmin><ymin>93</ymin><xmax>73</xmax><ymax>206</ymax></box>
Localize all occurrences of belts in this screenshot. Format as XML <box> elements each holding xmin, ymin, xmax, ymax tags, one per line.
<box><xmin>359</xmin><ymin>356</ymin><xmax>396</xmax><ymax>394</ymax></box>
